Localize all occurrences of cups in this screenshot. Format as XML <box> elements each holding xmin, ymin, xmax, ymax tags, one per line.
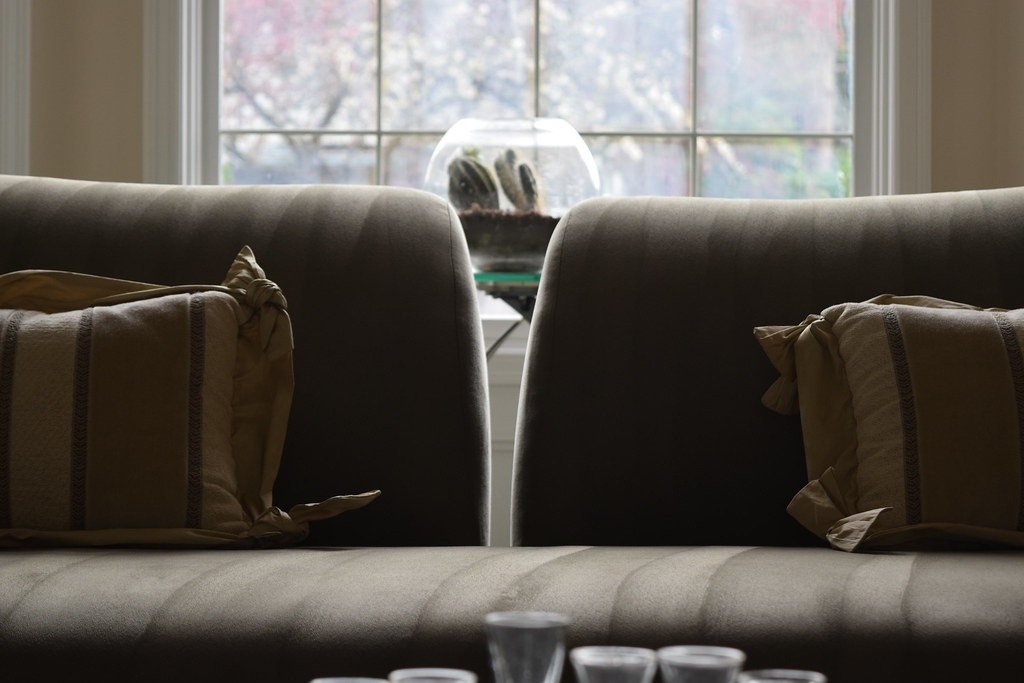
<box><xmin>313</xmin><ymin>607</ymin><xmax>826</xmax><ymax>683</ymax></box>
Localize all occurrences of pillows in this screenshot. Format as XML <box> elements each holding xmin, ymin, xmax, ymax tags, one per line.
<box><xmin>1</xmin><ymin>246</ymin><xmax>380</xmax><ymax>549</ymax></box>
<box><xmin>754</xmin><ymin>295</ymin><xmax>1024</xmax><ymax>552</ymax></box>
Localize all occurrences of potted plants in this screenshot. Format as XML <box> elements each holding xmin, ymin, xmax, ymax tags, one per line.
<box><xmin>424</xmin><ymin>117</ymin><xmax>601</xmax><ymax>271</ymax></box>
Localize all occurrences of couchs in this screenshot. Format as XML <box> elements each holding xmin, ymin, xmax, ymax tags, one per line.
<box><xmin>1</xmin><ymin>175</ymin><xmax>1024</xmax><ymax>683</ymax></box>
